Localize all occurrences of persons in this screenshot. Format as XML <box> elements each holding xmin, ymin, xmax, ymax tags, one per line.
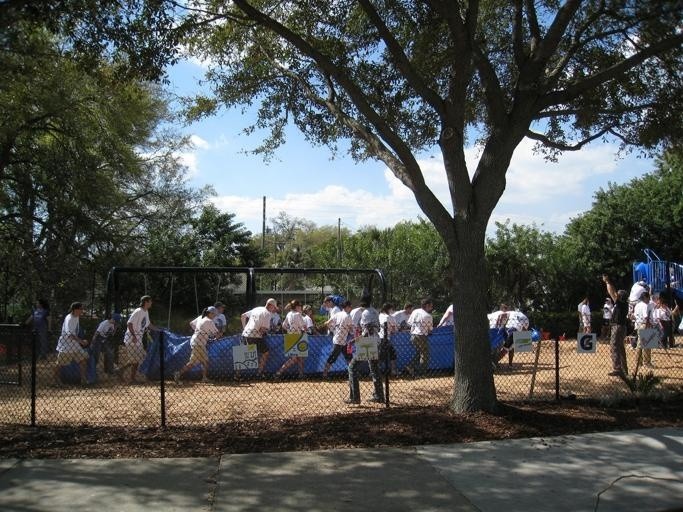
<box><xmin>23</xmin><ymin>299</ymin><xmax>51</xmax><ymax>360</ymax></box>
<box><xmin>342</xmin><ymin>297</ymin><xmax>384</xmax><ymax>403</ymax></box>
<box><xmin>115</xmin><ymin>295</ymin><xmax>161</xmax><ymax>384</ymax></box>
<box><xmin>47</xmin><ymin>301</ymin><xmax>94</xmax><ymax>388</ymax></box>
<box><xmin>213</xmin><ymin>300</ymin><xmax>227</xmax><ymax>337</ymax></box>
<box><xmin>600</xmin><ymin>272</ymin><xmax>628</xmax><ymax>376</ymax></box>
<box><xmin>232</xmin><ymin>298</ymin><xmax>277</xmax><ymax>380</ymax></box>
<box><xmin>633</xmin><ymin>291</ymin><xmax>657</xmax><ymax>368</ymax></box>
<box><xmin>269</xmin><ymin>295</ymin><xmax>530</xmax><ymax>381</ymax></box>
<box><xmin>87</xmin><ymin>313</ymin><xmax>119</xmax><ymax>374</ymax></box>
<box><xmin>173</xmin><ymin>306</ymin><xmax>217</xmax><ymax>384</ymax></box>
<box><xmin>578</xmin><ymin>282</ymin><xmax>678</xmax><ymax>349</ymax></box>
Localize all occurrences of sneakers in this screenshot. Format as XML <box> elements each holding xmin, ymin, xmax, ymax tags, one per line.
<box><xmin>344</xmin><ymin>398</ymin><xmax>359</xmax><ymax>404</ymax></box>
<box><xmin>365</xmin><ymin>397</ymin><xmax>384</xmax><ymax>403</ymax></box>
<box><xmin>113</xmin><ymin>367</ymin><xmax>125</xmax><ymax>384</ymax></box>
<box><xmin>174</xmin><ymin>371</ymin><xmax>180</xmax><ymax>385</ymax></box>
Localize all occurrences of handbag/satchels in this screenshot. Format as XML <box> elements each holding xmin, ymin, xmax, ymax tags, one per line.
<box><xmin>354</xmin><ymin>330</ymin><xmax>378</xmax><ymax>362</ymax></box>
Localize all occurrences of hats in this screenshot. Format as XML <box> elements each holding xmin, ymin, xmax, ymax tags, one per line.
<box><xmin>206</xmin><ymin>306</ymin><xmax>218</xmax><ymax>316</ymax></box>
<box><xmin>67</xmin><ymin>302</ymin><xmax>82</xmax><ymax>313</ymax></box>
<box><xmin>266</xmin><ymin>298</ymin><xmax>278</xmax><ymax>310</ymax></box>
<box><xmin>214</xmin><ymin>302</ymin><xmax>225</xmax><ymax>308</ymax></box>
<box><xmin>138</xmin><ymin>295</ymin><xmax>151</xmax><ymax>307</ymax></box>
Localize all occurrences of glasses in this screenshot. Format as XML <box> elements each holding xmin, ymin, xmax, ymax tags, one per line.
<box><xmin>297</xmin><ymin>305</ymin><xmax>302</xmax><ymax>307</ymax></box>
